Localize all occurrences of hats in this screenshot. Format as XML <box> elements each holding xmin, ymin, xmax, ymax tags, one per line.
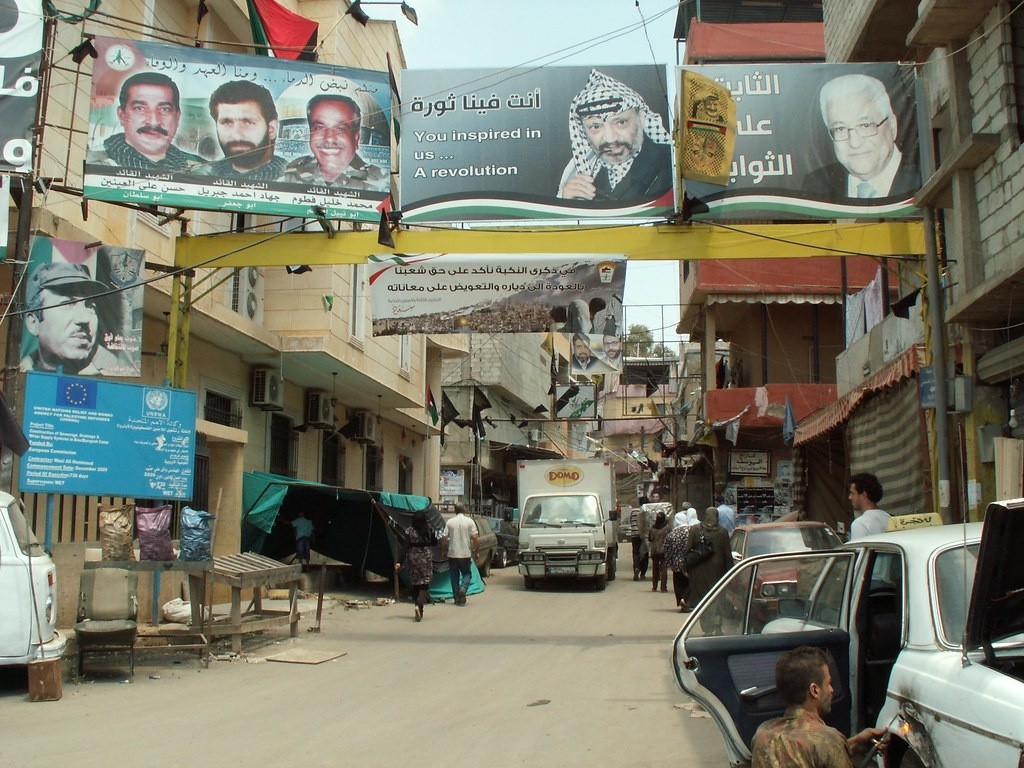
<box><xmin>25</xmin><ymin>261</ymin><xmax>110</xmax><ymax>306</ymax></box>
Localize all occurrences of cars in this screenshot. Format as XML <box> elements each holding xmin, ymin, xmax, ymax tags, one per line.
<box><xmin>720</xmin><ymin>518</ymin><xmax>844</xmax><ymax>622</ymax></box>
<box><xmin>670</xmin><ymin>497</ymin><xmax>1024</xmax><ymax>767</ymax></box>
<box><xmin>437</xmin><ymin>511</ymin><xmax>499</xmax><ymax>577</ymax></box>
<box><xmin>484</xmin><ymin>515</ymin><xmax>520</xmax><ymax>568</ymax></box>
<box><xmin>0</xmin><ymin>490</ymin><xmax>69</xmax><ymax>668</ymax></box>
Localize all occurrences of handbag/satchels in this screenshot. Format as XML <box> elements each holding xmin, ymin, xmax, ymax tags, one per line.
<box><xmin>686</xmin><ymin>523</ymin><xmax>716</xmax><ymax>571</ymax></box>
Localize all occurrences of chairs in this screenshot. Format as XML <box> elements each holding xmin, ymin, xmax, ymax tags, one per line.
<box><xmin>869</xmin><ymin>592</ymin><xmax>903</xmax><ymax>671</ymax></box>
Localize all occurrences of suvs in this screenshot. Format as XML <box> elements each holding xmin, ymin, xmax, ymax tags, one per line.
<box><xmin>617</xmin><ymin>503</ymin><xmax>634</xmax><ymax>543</ymax></box>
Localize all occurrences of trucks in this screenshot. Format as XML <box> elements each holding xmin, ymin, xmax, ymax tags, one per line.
<box><xmin>515</xmin><ymin>457</ymin><xmax>621</xmax><ymax>591</ymax></box>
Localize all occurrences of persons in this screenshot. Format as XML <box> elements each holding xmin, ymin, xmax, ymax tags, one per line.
<box><xmin>801</xmin><ymin>75</ymin><xmax>921</xmax><ymax>198</ymax></box>
<box><xmin>98</xmin><ymin>72</ymin><xmax>385</xmax><ymax>191</ymax></box>
<box><xmin>849</xmin><ymin>473</ymin><xmax>897</xmax><ymax>596</ymax></box>
<box><xmin>648</xmin><ymin>502</ymin><xmax>700</xmax><ymax>611</ymax></box>
<box><xmin>557</xmin><ymin>69</ymin><xmax>671</xmax><ymax>200</ymax></box>
<box><xmin>441</xmin><ymin>502</ymin><xmax>480</xmax><ymax>606</ymax></box>
<box><xmin>277</xmin><ymin>508</ymin><xmax>316</xmax><ymax>570</ymax></box>
<box><xmin>573</xmin><ymin>333</ymin><xmax>621</xmax><ymax>370</ymax></box>
<box><xmin>552</xmin><ymin>298</ymin><xmax>606</xmax><ymax>334</ymax></box>
<box><xmin>683</xmin><ymin>507</ymin><xmax>734</xmax><ymax>636</ymax></box>
<box><xmin>751</xmin><ymin>647</ymin><xmax>890</xmax><ymax>768</ymax></box>
<box><xmin>630</xmin><ymin>497</ymin><xmax>649</xmax><ymax>581</ymax></box>
<box><xmin>20</xmin><ymin>262</ymin><xmax>117</xmax><ymax>376</ymax></box>
<box><xmin>395</xmin><ymin>511</ymin><xmax>438</xmax><ymax>621</ymax></box>
<box><xmin>651</xmin><ymin>489</ymin><xmax>663</xmax><ymax>502</ymax></box>
<box><xmin>715</xmin><ymin>497</ymin><xmax>734</xmax><ymax>533</ymax></box>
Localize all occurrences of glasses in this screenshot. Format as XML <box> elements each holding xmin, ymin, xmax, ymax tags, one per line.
<box><xmin>827</xmin><ymin>116</ymin><xmax>890</xmax><ymax>142</ymax></box>
<box><xmin>603</xmin><ymin>341</ymin><xmax>620</xmax><ymax>347</ymax></box>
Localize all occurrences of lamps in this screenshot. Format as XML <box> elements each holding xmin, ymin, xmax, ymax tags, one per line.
<box><xmin>376</xmin><ymin>395</ymin><xmax>382</xmax><ymax>424</ymax></box>
<box><xmin>359</xmin><ymin>0</ymin><xmax>418</xmax><ymax>26</ymax></box>
<box><xmin>331</xmin><ymin>371</ymin><xmax>340</xmax><ymax>407</ymax></box>
<box><xmin>412</xmin><ymin>424</ymin><xmax>417</xmax><ymax>448</ymax></box>
<box><xmin>953</xmin><ymin>373</ymin><xmax>974</xmax><ymax>412</ymax></box>
<box><xmin>683</xmin><ymin>195</ymin><xmax>711</xmax><ymax>217</ymax></box>
<box><xmin>388</xmin><ymin>210</ymin><xmax>403</xmax><ymax>231</ymax></box>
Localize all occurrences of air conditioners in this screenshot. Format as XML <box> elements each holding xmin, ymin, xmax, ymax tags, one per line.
<box><xmin>306</xmin><ymin>392</ymin><xmax>335</xmax><ymax>425</ymax></box>
<box><xmin>235</xmin><ymin>260</ymin><xmax>269</xmax><ymax>291</ymax></box>
<box><xmin>355</xmin><ymin>410</ymin><xmax>378</xmax><ymax>440</ymax></box>
<box><xmin>250</xmin><ymin>368</ymin><xmax>286</xmax><ymax>407</ymax></box>
<box><xmin>232</xmin><ymin>289</ymin><xmax>264</xmax><ymax>322</ymax></box>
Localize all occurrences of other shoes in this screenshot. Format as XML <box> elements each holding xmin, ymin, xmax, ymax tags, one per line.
<box><xmin>652</xmin><ymin>588</ymin><xmax>656</xmax><ymax>592</ymax></box>
<box><xmin>680</xmin><ymin>598</ymin><xmax>689</xmax><ymax>612</ymax></box>
<box><xmin>453</xmin><ymin>589</ymin><xmax>467</xmax><ymax>607</ymax></box>
<box><xmin>415</xmin><ymin>605</ymin><xmax>423</xmax><ymax>622</ymax></box>
<box><xmin>633</xmin><ymin>568</ymin><xmax>640</xmax><ymax>581</ymax></box>
<box><xmin>662</xmin><ymin>590</ymin><xmax>668</xmax><ymax>593</ymax></box>
<box><xmin>640</xmin><ymin>574</ymin><xmax>645</xmax><ymax>579</ymax></box>
